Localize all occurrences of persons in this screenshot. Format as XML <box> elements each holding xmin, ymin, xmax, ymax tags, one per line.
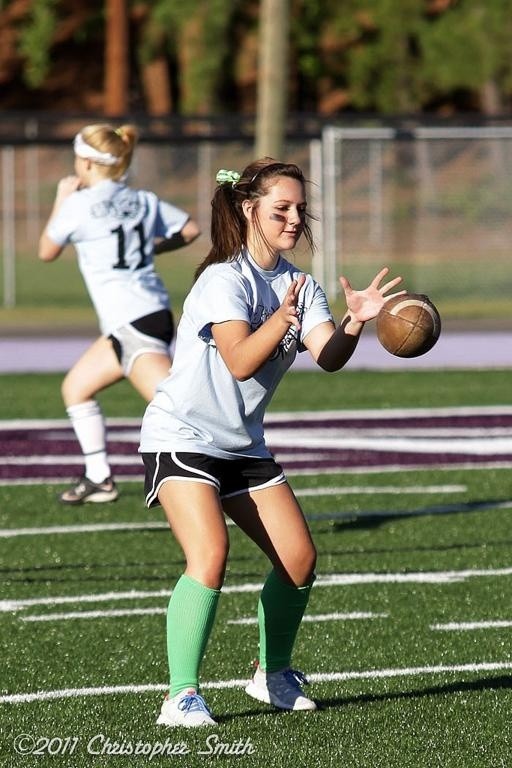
<box><xmin>37</xmin><ymin>124</ymin><xmax>200</xmax><ymax>505</ymax></box>
<box><xmin>138</xmin><ymin>156</ymin><xmax>407</xmax><ymax>729</ymax></box>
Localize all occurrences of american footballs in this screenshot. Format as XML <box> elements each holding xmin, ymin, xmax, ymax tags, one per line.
<box><xmin>377</xmin><ymin>292</ymin><xmax>441</xmax><ymax>357</ymax></box>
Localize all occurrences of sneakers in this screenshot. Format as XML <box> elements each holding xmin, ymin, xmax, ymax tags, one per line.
<box><xmin>243</xmin><ymin>665</ymin><xmax>315</xmax><ymax>712</ymax></box>
<box><xmin>61</xmin><ymin>478</ymin><xmax>118</xmax><ymax>502</ymax></box>
<box><xmin>152</xmin><ymin>687</ymin><xmax>220</xmax><ymax>730</ymax></box>
